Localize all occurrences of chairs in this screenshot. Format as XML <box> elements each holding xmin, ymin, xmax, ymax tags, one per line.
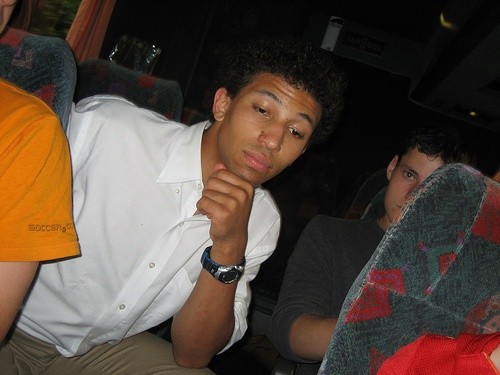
<box><xmin>317</xmin><ymin>165</ymin><xmax>500</xmax><ymax>375</ymax></box>
<box><xmin>345</xmin><ymin>167</ymin><xmax>385</xmax><ymax>221</ymax></box>
<box><xmin>0</xmin><ymin>28</ymin><xmax>76</xmax><ymax>133</ymax></box>
<box><xmin>73</xmin><ymin>57</ymin><xmax>183</xmax><ymax>121</ymax></box>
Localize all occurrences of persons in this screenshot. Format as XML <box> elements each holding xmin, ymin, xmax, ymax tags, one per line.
<box><xmin>269</xmin><ymin>126</ymin><xmax>500</xmax><ymax>375</ymax></box>
<box><xmin>0</xmin><ymin>37</ymin><xmax>344</xmax><ymax>375</ymax></box>
<box><xmin>0</xmin><ymin>0</ymin><xmax>80</xmax><ymax>375</ymax></box>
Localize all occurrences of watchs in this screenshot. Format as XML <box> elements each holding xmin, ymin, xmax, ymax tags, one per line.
<box><xmin>202</xmin><ymin>247</ymin><xmax>246</xmax><ymax>283</ymax></box>
